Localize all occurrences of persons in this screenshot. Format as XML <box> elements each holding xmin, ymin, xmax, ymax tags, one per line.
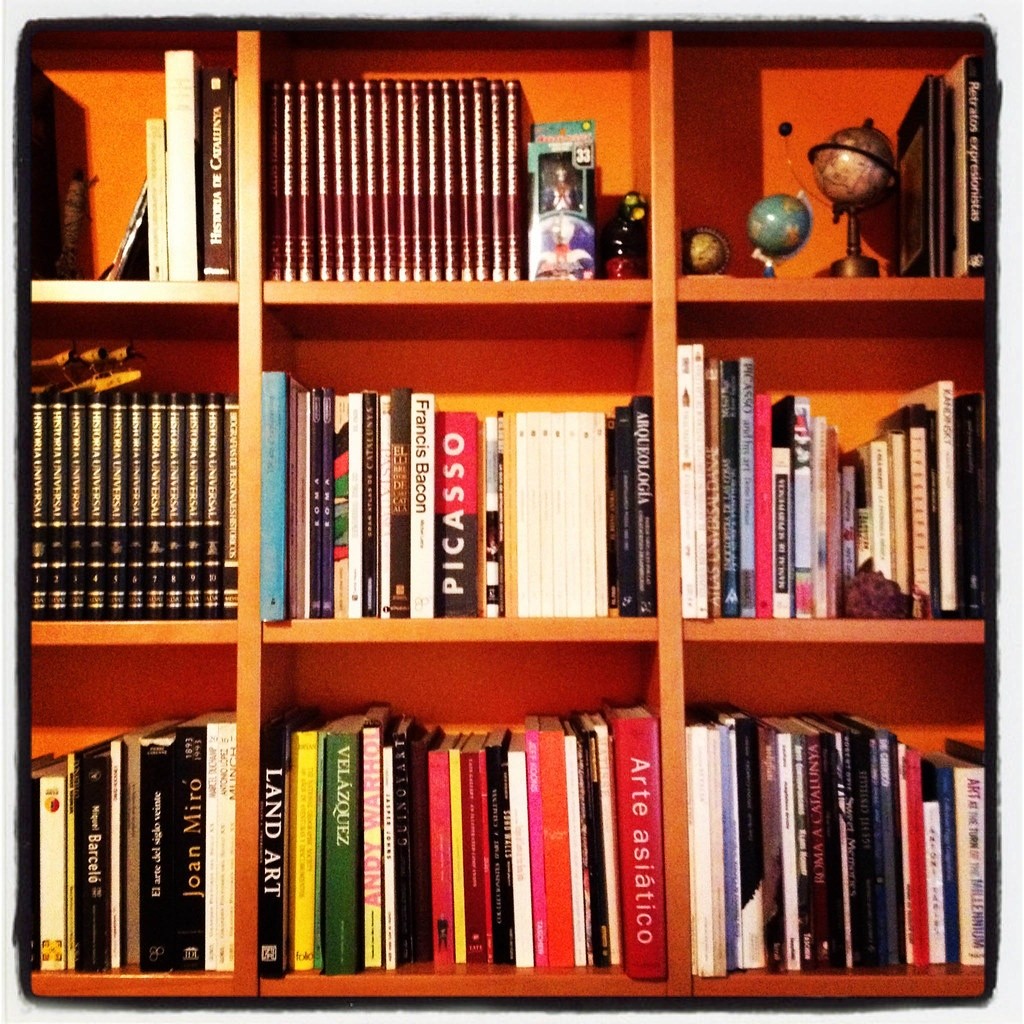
<box><xmin>542</xmin><ymin>163</ymin><xmax>584</xmax><ymax>212</ymax></box>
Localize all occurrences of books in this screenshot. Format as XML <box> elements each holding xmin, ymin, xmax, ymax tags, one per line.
<box><xmin>31</xmin><ymin>710</ymin><xmax>234</xmax><ymax>972</ymax></box>
<box><xmin>895</xmin><ymin>54</ymin><xmax>984</xmax><ymax>276</ymax></box>
<box><xmin>260</xmin><ymin>371</ymin><xmax>658</xmax><ymax>621</ymax></box>
<box><xmin>31</xmin><ymin>48</ymin><xmax>237</xmax><ymax>281</ymax></box>
<box><xmin>32</xmin><ymin>392</ymin><xmax>239</xmax><ymax>619</ymax></box>
<box><xmin>263</xmin><ymin>77</ymin><xmax>535</xmax><ymax>283</ymax></box>
<box><xmin>256</xmin><ymin>703</ymin><xmax>666</xmax><ymax>979</ymax></box>
<box><xmin>676</xmin><ymin>344</ymin><xmax>984</xmax><ymax>620</ymax></box>
<box><xmin>685</xmin><ymin>704</ymin><xmax>986</xmax><ymax>977</ymax></box>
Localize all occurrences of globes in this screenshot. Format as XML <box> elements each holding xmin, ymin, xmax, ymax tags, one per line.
<box><xmin>808</xmin><ymin>118</ymin><xmax>900</xmax><ymax>277</ymax></box>
<box><xmin>747</xmin><ymin>191</ymin><xmax>811</xmax><ymax>278</ymax></box>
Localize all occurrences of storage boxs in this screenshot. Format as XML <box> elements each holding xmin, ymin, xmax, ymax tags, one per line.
<box><xmin>528</xmin><ymin>118</ymin><xmax>597</xmax><ymax>280</ymax></box>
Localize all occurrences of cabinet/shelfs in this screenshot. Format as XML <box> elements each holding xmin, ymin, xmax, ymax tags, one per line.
<box><xmin>32</xmin><ymin>26</ymin><xmax>990</xmax><ymax>1000</ymax></box>
